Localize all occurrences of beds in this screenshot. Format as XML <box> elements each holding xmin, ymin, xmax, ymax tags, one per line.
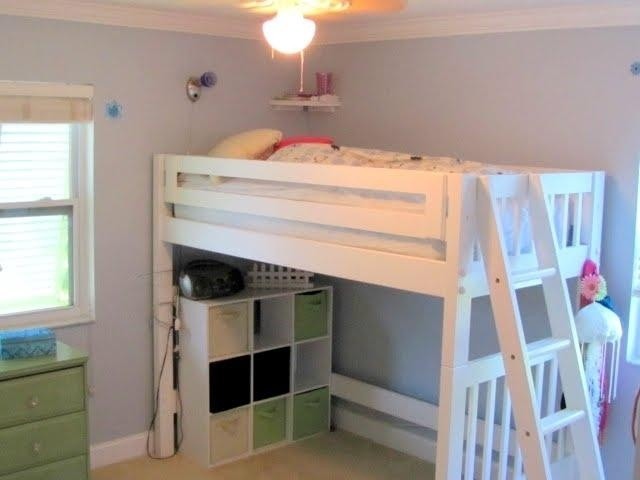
<box><xmin>142</xmin><ymin>130</ymin><xmax>615</xmax><ymax>479</ymax></box>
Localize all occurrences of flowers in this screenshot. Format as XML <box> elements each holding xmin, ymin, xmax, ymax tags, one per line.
<box><xmin>580</xmin><ymin>274</ymin><xmax>612</xmax><ymax>305</ymax></box>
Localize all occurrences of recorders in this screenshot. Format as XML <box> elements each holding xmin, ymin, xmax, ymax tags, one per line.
<box><xmin>179</xmin><ymin>259</ymin><xmax>245</xmax><ymax>300</ymax></box>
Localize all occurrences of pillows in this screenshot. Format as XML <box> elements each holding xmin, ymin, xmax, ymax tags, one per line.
<box><xmin>204</xmin><ymin>126</ymin><xmax>283</xmax><ymax>183</ymax></box>
<box><xmin>274</xmin><ymin>133</ymin><xmax>335</xmax><ymax>150</ymax></box>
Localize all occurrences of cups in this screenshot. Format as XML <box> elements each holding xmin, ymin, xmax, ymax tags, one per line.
<box><xmin>314</xmin><ymin>71</ymin><xmax>332</xmax><ymax>97</ymax></box>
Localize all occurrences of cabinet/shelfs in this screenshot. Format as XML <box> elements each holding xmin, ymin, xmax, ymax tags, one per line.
<box><xmin>1</xmin><ymin>333</ymin><xmax>94</xmax><ymax>478</ymax></box>
<box><xmin>175</xmin><ymin>281</ymin><xmax>336</xmax><ymax>471</ymax></box>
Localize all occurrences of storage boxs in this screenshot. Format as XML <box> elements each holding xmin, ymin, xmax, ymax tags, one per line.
<box><xmin>1</xmin><ymin>326</ymin><xmax>59</xmax><ymax>361</ymax></box>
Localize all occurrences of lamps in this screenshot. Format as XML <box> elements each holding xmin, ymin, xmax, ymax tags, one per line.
<box><xmin>262</xmin><ymin>3</ymin><xmax>318</xmax><ymax>56</ymax></box>
<box><xmin>185</xmin><ymin>68</ymin><xmax>218</xmax><ymax>103</ymax></box>
